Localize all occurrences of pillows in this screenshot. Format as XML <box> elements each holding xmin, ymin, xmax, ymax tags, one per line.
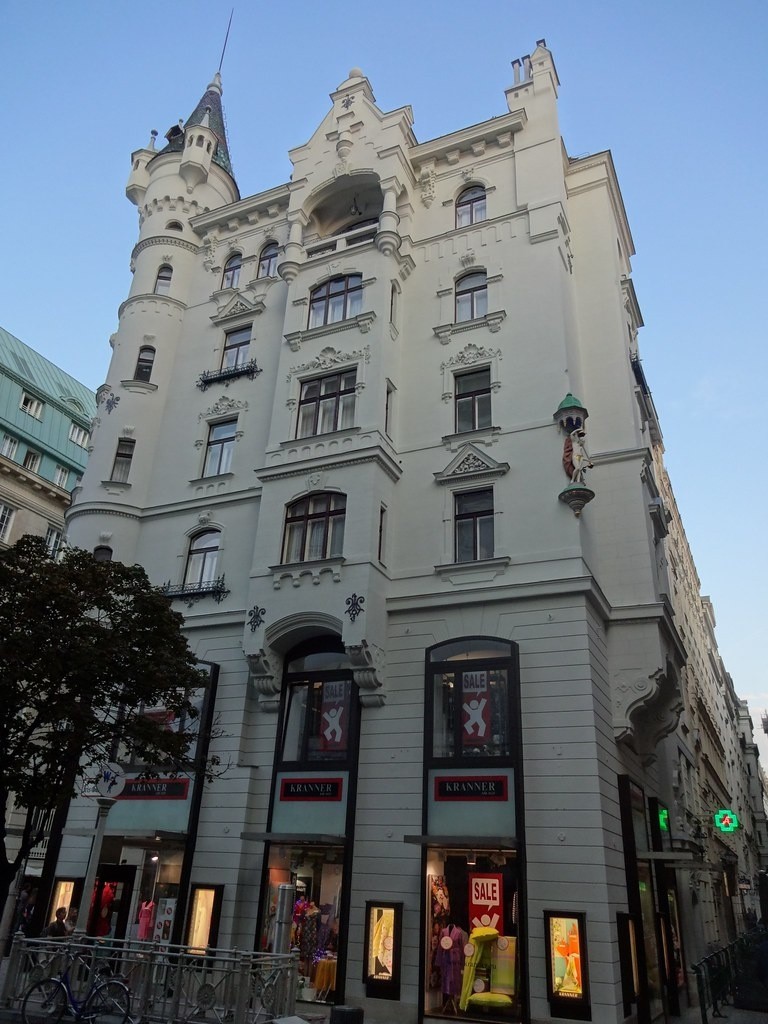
<box><xmin>471</xmin><ymin>927</ymin><xmax>499</xmax><ymax>941</ymax></box>
<box><xmin>469</xmin><ymin>992</ymin><xmax>512</xmax><ymax>1007</ymax></box>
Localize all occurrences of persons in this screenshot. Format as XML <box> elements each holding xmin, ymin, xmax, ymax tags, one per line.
<box><xmin>743</xmin><ymin>907</ymin><xmax>755</xmax><ymax>933</ymax></box>
<box><xmin>301</xmin><ymin>904</ymin><xmax>338</xmax><ymax>987</ymax></box>
<box><xmin>435</xmin><ymin>924</ymin><xmax>465</xmax><ymax>996</ymax></box>
<box><xmin>570</xmin><ymin>428</ymin><xmax>593</xmax><ymax>483</ymax></box>
<box><xmin>40</xmin><ymin>907</ymin><xmax>78</xmax><ymax>978</ymax></box>
<box><xmin>155</xmin><ymin>907</ymin><xmax>172</xmax><ymax>943</ymax></box>
<box><xmin>433</xmin><ymin>875</ymin><xmax>450</xmax><ymax>924</ymax></box>
<box><xmin>297</xmin><ymin>902</ymin><xmax>322</xmax><ymax>979</ymax></box>
<box><xmin>291</xmin><ymin>896</ymin><xmax>308</xmax><ymax>948</ymax></box>
<box><xmin>139</xmin><ymin>896</ymin><xmax>155</xmax><ymax>950</ymax></box>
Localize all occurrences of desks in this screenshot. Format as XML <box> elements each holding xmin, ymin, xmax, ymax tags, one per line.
<box><xmin>315</xmin><ymin>958</ymin><xmax>338</xmax><ymax>1002</ymax></box>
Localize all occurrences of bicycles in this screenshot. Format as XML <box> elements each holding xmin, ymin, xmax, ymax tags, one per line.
<box><xmin>21</xmin><ymin>948</ymin><xmax>131</xmax><ymax>1024</ymax></box>
